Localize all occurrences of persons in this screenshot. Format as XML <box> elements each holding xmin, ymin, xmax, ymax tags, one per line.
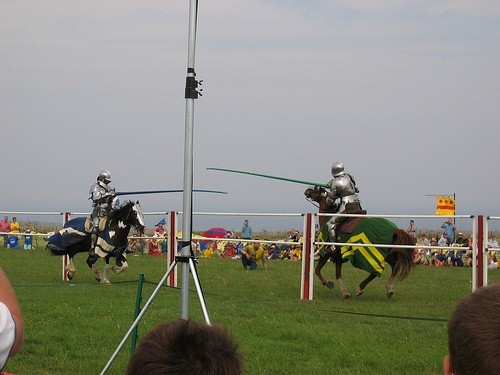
<box><xmin>443</xmin><ymin>282</ymin><xmax>500</xmax><ymax>375</ymax></box>
<box><xmin>325</xmin><ymin>162</ymin><xmax>362</xmax><ymax>252</ymax></box>
<box><xmin>0</xmin><ymin>216</ymin><xmax>36</xmax><ymax>250</ymax></box>
<box><xmin>89</xmin><ymin>169</ymin><xmax>116</xmax><ymax>251</ymax></box>
<box><xmin>126</xmin><ymin>318</ymin><xmax>243</xmax><ymax>375</ymax></box>
<box><xmin>124</xmin><ymin>220</ymin><xmax>500</xmax><ymax>269</ymax></box>
<box><xmin>241</xmin><ymin>239</ymin><xmax>268</xmax><ymax>271</ymax></box>
<box><xmin>0</xmin><ymin>266</ymin><xmax>24</xmax><ymax>375</ymax></box>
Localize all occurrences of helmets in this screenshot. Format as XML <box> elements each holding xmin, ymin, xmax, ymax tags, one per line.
<box><xmin>97</xmin><ymin>169</ymin><xmax>111</xmax><ymax>185</ymax></box>
<box><xmin>331</xmin><ymin>162</ymin><xmax>346</xmax><ymax>178</ymax></box>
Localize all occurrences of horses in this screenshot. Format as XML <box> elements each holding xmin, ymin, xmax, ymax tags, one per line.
<box><xmin>43</xmin><ymin>200</ymin><xmax>145</xmax><ymax>285</ymax></box>
<box><xmin>304</xmin><ymin>185</ymin><xmax>417</xmax><ymax>300</ymax></box>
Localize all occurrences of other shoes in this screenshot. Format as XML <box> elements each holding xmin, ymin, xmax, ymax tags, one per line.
<box><xmin>324</xmin><ymin>245</ymin><xmax>337</xmax><ymax>252</ymax></box>
<box><xmin>89</xmin><ymin>247</ymin><xmax>96</xmax><ymax>257</ymax></box>
